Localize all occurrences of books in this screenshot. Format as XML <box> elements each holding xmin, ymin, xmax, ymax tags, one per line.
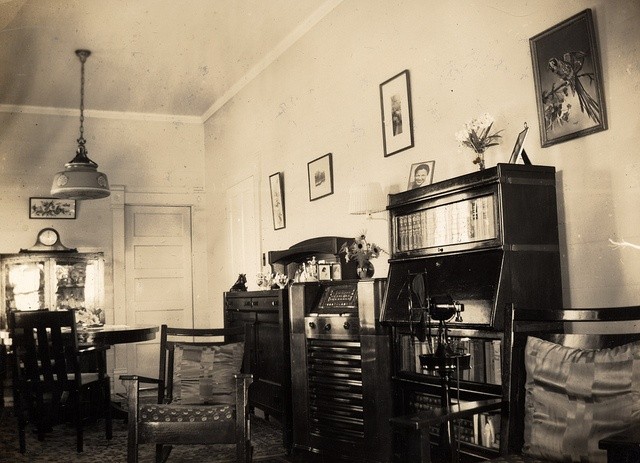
<box><xmin>395</xmin><ymin>192</ymin><xmax>496</xmax><ymax>252</ymax></box>
<box><xmin>403</xmin><ymin>389</ymin><xmax>499</xmax><ymax>450</ymax></box>
<box><xmin>398</xmin><ymin>332</ymin><xmax>502</xmax><ymax>388</ymax></box>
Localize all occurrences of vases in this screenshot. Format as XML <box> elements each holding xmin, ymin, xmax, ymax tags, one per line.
<box><xmin>355</xmin><ymin>258</ymin><xmax>375</xmax><ymax>280</ymax></box>
<box><xmin>478</xmin><ymin>150</ymin><xmax>485</xmax><ymax>171</ymax></box>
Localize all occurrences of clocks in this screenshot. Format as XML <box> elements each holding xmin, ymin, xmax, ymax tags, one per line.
<box><xmin>36</xmin><ymin>228</ymin><xmax>60</xmax><ymax>247</ymax></box>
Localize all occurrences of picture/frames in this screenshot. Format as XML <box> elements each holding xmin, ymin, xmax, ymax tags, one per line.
<box><xmin>316</xmin><ymin>263</ymin><xmax>331</xmax><ymax>282</ymax></box>
<box><xmin>379</xmin><ymin>68</ymin><xmax>415</xmax><ymax>158</ymax></box>
<box><xmin>529</xmin><ymin>7</ymin><xmax>609</xmax><ymax>148</ymax></box>
<box><xmin>307</xmin><ymin>152</ymin><xmax>334</xmax><ymax>201</ymax></box>
<box><xmin>331</xmin><ymin>262</ymin><xmax>343</xmax><ymax>281</ymax></box>
<box><xmin>268</xmin><ymin>171</ymin><xmax>286</xmax><ymax>231</ymax></box>
<box><xmin>406</xmin><ymin>160</ymin><xmax>436</xmax><ymax>192</ymax></box>
<box><xmin>510</xmin><ymin>126</ymin><xmax>529</xmax><ymax>165</ymax></box>
<box><xmin>28</xmin><ymin>196</ymin><xmax>77</xmax><ymax>220</ymax></box>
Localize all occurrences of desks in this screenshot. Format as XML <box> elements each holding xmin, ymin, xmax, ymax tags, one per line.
<box><xmin>1</xmin><ymin>325</ymin><xmax>160</xmax><ymax>441</ymax></box>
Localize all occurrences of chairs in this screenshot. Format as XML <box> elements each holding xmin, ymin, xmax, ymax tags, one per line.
<box><xmin>9</xmin><ymin>309</ymin><xmax>113</xmax><ymax>454</ymax></box>
<box><xmin>118</xmin><ymin>322</ymin><xmax>260</xmax><ymax>462</ymax></box>
<box><xmin>483</xmin><ymin>299</ymin><xmax>640</xmax><ymax>463</ymax></box>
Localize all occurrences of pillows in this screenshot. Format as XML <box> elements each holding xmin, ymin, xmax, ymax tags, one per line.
<box><xmin>172</xmin><ymin>342</ymin><xmax>245</xmax><ymax>407</ymax></box>
<box><xmin>520</xmin><ymin>336</ymin><xmax>640</xmax><ymax>463</ymax></box>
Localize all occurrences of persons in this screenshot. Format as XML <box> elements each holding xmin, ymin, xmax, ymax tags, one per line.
<box><xmin>407</xmin><ymin>162</ymin><xmax>429</xmax><ymax>190</ymax></box>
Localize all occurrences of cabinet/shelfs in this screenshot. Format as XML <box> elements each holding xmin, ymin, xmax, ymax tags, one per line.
<box><xmin>0</xmin><ymin>251</ymin><xmax>104</xmax><ymax>330</ymax></box>
<box><xmin>224</xmin><ymin>292</ymin><xmax>285</xmax><ymax>389</ymax></box>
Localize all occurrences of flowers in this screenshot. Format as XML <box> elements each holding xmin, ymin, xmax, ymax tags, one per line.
<box><xmin>339</xmin><ymin>229</ymin><xmax>389</xmax><ymax>272</ymax></box>
<box><xmin>455</xmin><ymin>108</ymin><xmax>504</xmax><ymax>165</ymax></box>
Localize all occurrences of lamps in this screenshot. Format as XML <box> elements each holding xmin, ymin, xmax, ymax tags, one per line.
<box><xmin>48</xmin><ymin>48</ymin><xmax>111</xmax><ymax>203</ymax></box>
